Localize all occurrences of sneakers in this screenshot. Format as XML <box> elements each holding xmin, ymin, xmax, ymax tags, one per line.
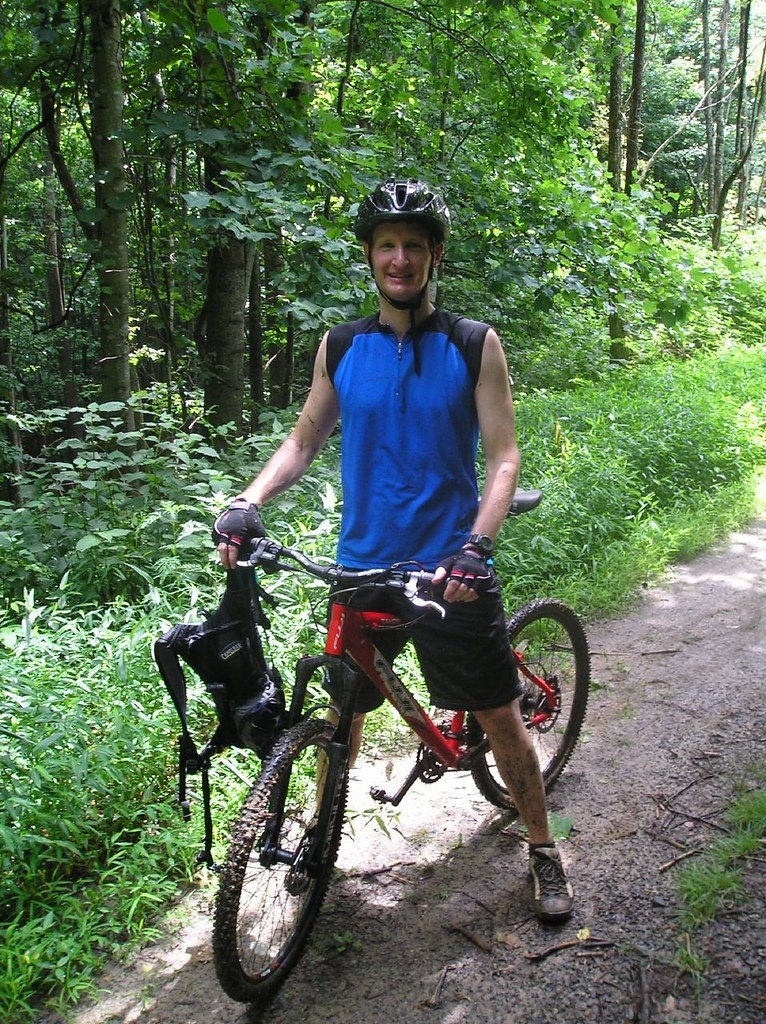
<box><xmin>530</xmin><ymin>842</ymin><xmax>575</xmax><ymax>921</ymax></box>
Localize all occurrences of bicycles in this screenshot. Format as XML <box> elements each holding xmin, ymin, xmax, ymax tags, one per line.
<box><xmin>210</xmin><ymin>486</ymin><xmax>591</xmax><ymax>1003</ymax></box>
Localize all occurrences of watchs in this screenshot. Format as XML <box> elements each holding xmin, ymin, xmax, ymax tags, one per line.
<box><xmin>465</xmin><ymin>534</ymin><xmax>494</xmax><ymax>554</ymax></box>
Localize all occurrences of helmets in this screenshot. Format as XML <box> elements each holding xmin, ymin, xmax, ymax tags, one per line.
<box><xmin>354</xmin><ymin>178</ymin><xmax>453</xmax><ymax>244</ymax></box>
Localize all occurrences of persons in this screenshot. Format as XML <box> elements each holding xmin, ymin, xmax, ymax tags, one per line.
<box><xmin>211</xmin><ymin>179</ymin><xmax>575</xmax><ymax>921</ymax></box>
